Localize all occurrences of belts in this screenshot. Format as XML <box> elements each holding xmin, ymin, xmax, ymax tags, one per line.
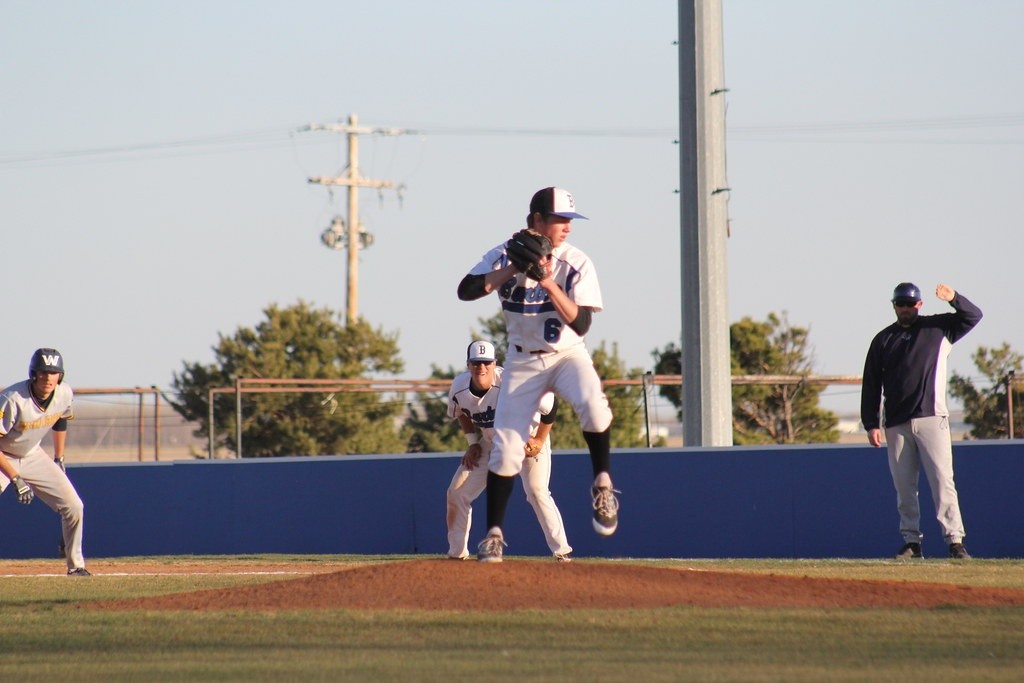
<box><xmin>515</xmin><ymin>345</ymin><xmax>549</xmax><ymax>354</ymax></box>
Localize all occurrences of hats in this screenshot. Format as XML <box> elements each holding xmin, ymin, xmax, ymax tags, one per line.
<box><xmin>891</xmin><ymin>282</ymin><xmax>920</xmax><ymax>300</ymax></box>
<box><xmin>530</xmin><ymin>187</ymin><xmax>589</xmax><ymax>220</ymax></box>
<box><xmin>466</xmin><ymin>341</ymin><xmax>497</xmax><ymax>362</ymax></box>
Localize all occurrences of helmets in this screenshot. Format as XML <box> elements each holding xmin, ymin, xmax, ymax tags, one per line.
<box><xmin>29</xmin><ymin>347</ymin><xmax>65</xmax><ymax>384</ymax></box>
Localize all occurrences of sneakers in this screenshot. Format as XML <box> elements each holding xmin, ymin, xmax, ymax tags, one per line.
<box><xmin>896</xmin><ymin>543</ymin><xmax>925</xmax><ymax>559</ymax></box>
<box><xmin>950</xmin><ymin>543</ymin><xmax>970</xmax><ymax>559</ymax></box>
<box><xmin>590</xmin><ymin>486</ymin><xmax>622</xmax><ymax>535</ymax></box>
<box><xmin>477</xmin><ymin>535</ymin><xmax>508</xmax><ymax>562</ymax></box>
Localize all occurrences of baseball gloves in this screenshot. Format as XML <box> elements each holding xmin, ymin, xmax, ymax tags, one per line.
<box><xmin>523</xmin><ymin>434</ymin><xmax>543</xmax><ymax>462</ymax></box>
<box><xmin>502</xmin><ymin>227</ymin><xmax>555</xmax><ymax>281</ymax></box>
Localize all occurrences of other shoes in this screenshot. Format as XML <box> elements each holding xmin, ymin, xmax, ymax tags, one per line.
<box><xmin>67</xmin><ymin>568</ymin><xmax>91</xmax><ymax>576</ymax></box>
<box><xmin>555</xmin><ymin>555</ymin><xmax>570</xmax><ymax>561</ymax></box>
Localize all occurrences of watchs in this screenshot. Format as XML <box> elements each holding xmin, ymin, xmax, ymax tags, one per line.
<box><xmin>54</xmin><ymin>456</ymin><xmax>64</xmax><ymax>462</ymax></box>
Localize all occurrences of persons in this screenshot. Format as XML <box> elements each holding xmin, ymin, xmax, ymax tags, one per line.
<box><xmin>1</xmin><ymin>349</ymin><xmax>92</xmax><ymax>577</ymax></box>
<box><xmin>861</xmin><ymin>283</ymin><xmax>983</xmax><ymax>560</ymax></box>
<box><xmin>458</xmin><ymin>186</ymin><xmax>620</xmax><ymax>560</ymax></box>
<box><xmin>445</xmin><ymin>341</ymin><xmax>571</xmax><ymax>560</ymax></box>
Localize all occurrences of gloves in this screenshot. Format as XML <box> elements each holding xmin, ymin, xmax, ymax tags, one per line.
<box><xmin>11</xmin><ymin>475</ymin><xmax>34</xmax><ymax>504</ymax></box>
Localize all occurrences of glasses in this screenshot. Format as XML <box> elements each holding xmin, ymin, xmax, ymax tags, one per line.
<box><xmin>894</xmin><ymin>299</ymin><xmax>919</xmax><ymax>307</ymax></box>
<box><xmin>470</xmin><ymin>360</ymin><xmax>493</xmax><ymax>366</ymax></box>
